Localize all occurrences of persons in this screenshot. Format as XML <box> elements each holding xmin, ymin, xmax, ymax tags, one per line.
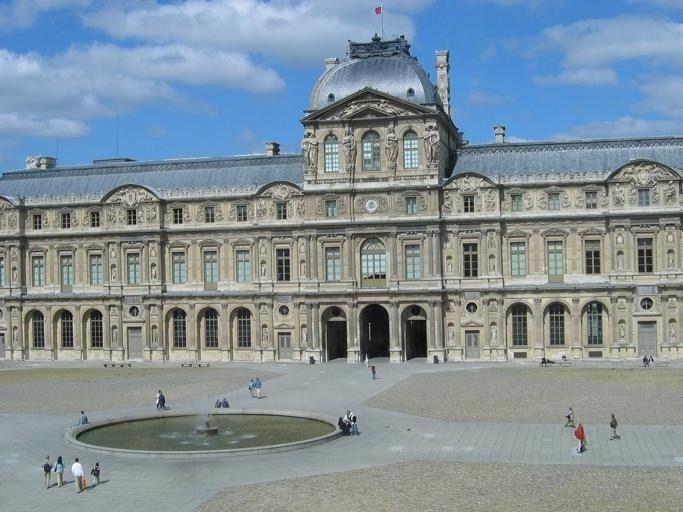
<box><xmin>44</xmin><ymin>456</ymin><xmax>101</xmax><ymax>494</ymax></box>
<box><xmin>541</xmin><ymin>357</ymin><xmax>546</xmax><ymax>366</ymax></box>
<box><xmin>385</xmin><ymin>124</ymin><xmax>398</xmax><ymax>171</ymax></box>
<box><xmin>372</xmin><ymin>364</ymin><xmax>376</xmax><ymax>379</ymax></box>
<box><xmin>154</xmin><ymin>390</ymin><xmax>166</xmax><ymax>410</ymax></box>
<box><xmin>249</xmin><ymin>377</ymin><xmax>262</xmax><ymax>399</ymax></box>
<box><xmin>564</xmin><ymin>407</ymin><xmax>576</xmax><ymax>428</ymax></box>
<box><xmin>300</xmin><ymin>124</ymin><xmax>318</xmax><ymax>173</ymax></box>
<box><xmin>574</xmin><ymin>423</ymin><xmax>585</xmax><ymax>452</ymax></box>
<box><xmin>643</xmin><ymin>355</ymin><xmax>654</xmax><ymax>368</ymax></box>
<box><xmin>608</xmin><ymin>414</ymin><xmax>621</xmax><ymax>440</ymax></box>
<box><xmin>0</xmin><ymin>184</ymin><xmax>676</xmax><ymax>231</ymax></box>
<box><xmin>343</xmin><ymin>127</ymin><xmax>356</xmax><ymax>172</ymax></box>
<box><xmin>78</xmin><ymin>410</ymin><xmax>88</xmax><ymax>425</ymax></box>
<box><xmin>422</xmin><ymin>125</ymin><xmax>441</xmax><ymax>166</ymax></box>
<box><xmin>215</xmin><ymin>398</ymin><xmax>228</xmax><ymax>408</ymax></box>
<box><xmin>338</xmin><ymin>410</ymin><xmax>359</xmax><ymax>435</ymax></box>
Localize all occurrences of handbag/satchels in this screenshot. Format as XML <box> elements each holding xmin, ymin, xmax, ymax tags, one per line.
<box><xmin>81</xmin><ymin>480</ymin><xmax>86</xmax><ymax>489</ymax></box>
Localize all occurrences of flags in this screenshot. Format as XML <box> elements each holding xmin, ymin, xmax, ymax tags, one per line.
<box><xmin>375</xmin><ymin>6</ymin><xmax>381</xmax><ymax>14</ymax></box>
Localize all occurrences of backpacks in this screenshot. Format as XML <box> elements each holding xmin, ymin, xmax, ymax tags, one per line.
<box><xmin>44</xmin><ymin>464</ymin><xmax>51</xmax><ymax>472</ymax></box>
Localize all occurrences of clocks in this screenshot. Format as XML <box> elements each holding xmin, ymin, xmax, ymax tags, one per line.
<box><xmin>379</xmin><ymin>199</ymin><xmax>385</xmax><ymax>213</ymax></box>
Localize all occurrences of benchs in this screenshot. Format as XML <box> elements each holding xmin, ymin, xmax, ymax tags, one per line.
<box><xmin>180</xmin><ymin>363</ymin><xmax>209</xmax><ymax>367</ymax></box>
<box><xmin>540</xmin><ymin>363</ymin><xmax>577</xmax><ymax>367</ymax></box>
<box><xmin>635</xmin><ymin>361</ymin><xmax>671</xmax><ymax>368</ymax></box>
<box><xmin>103</xmin><ymin>363</ymin><xmax>131</xmax><ymax>367</ymax></box>
